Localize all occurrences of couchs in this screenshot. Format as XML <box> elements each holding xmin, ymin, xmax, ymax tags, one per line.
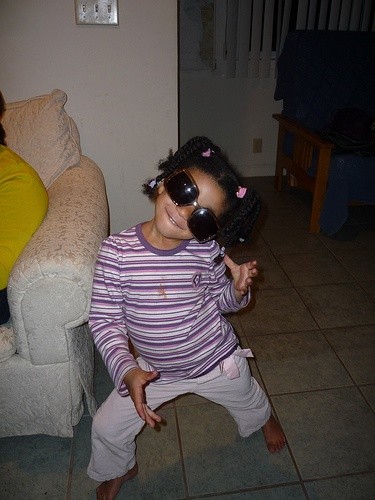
<box><xmin>0</xmin><ymin>154</ymin><xmax>115</xmax><ymax>439</ymax></box>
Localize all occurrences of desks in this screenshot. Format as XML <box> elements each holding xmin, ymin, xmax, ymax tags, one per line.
<box><xmin>270</xmin><ymin>114</ymin><xmax>375</xmax><ymax>235</ymax></box>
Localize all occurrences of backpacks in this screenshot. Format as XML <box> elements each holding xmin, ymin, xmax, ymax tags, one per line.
<box><xmin>318</xmin><ymin>106</ymin><xmax>375</xmax><ymax>157</ymax></box>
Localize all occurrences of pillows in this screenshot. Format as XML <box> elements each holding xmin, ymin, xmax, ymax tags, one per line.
<box><xmin>1</xmin><ymin>90</ymin><xmax>84</xmax><ymax>192</ymax></box>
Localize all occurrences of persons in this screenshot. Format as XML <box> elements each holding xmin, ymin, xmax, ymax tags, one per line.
<box><xmin>0</xmin><ymin>90</ymin><xmax>49</xmax><ymax>325</ymax></box>
<box><xmin>87</xmin><ymin>137</ymin><xmax>286</xmax><ymax>500</ymax></box>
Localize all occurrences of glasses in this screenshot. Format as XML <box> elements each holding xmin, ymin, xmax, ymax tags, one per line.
<box><xmin>163</xmin><ymin>168</ymin><xmax>219</xmax><ymax>244</ymax></box>
